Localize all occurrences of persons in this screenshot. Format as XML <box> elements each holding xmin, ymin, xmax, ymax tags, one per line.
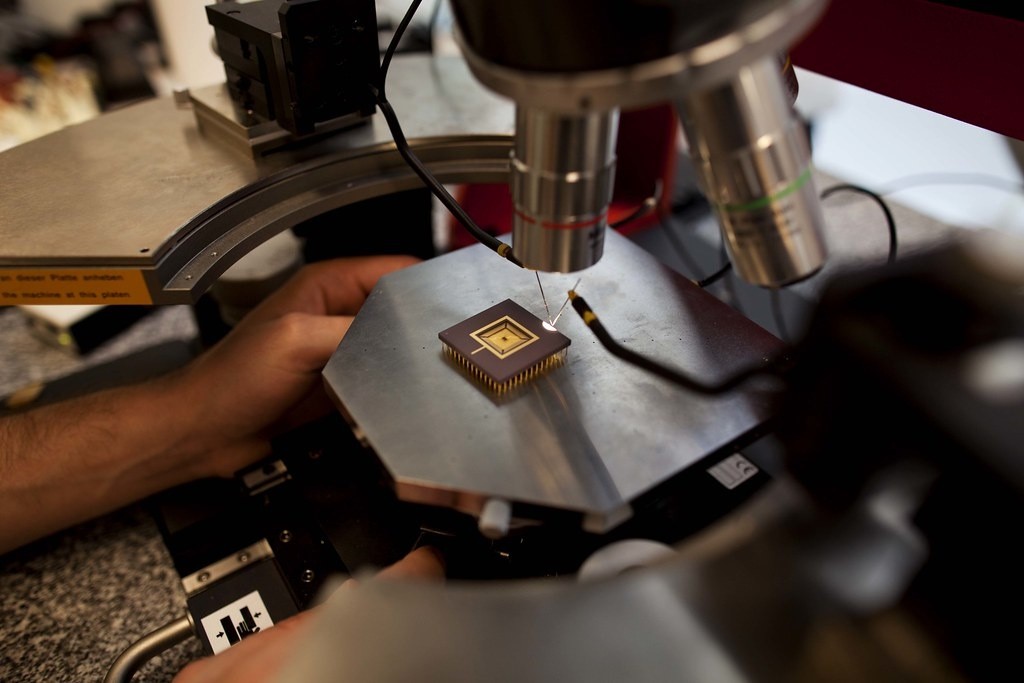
<box><xmin>0</xmin><ymin>254</ymin><xmax>447</xmax><ymax>682</ymax></box>
